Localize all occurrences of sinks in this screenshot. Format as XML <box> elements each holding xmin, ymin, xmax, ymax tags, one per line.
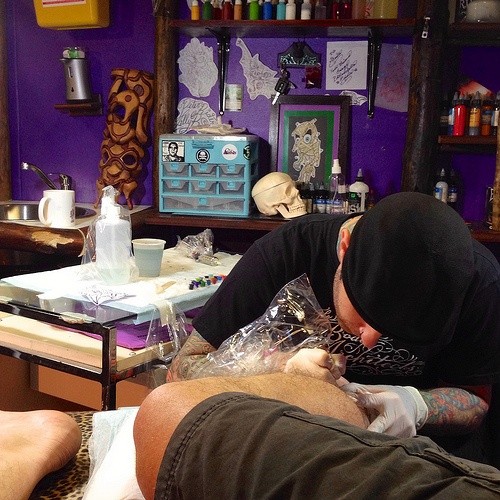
<box><xmin>0</xmin><ymin>203</ymin><xmax>89</xmax><ymax>220</ymax></box>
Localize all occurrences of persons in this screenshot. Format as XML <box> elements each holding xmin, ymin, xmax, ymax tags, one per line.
<box><xmin>0</xmin><ymin>375</ymin><xmax>499</xmax><ymax>500</ymax></box>
<box><xmin>167</xmin><ymin>193</ymin><xmax>500</xmax><ymax>438</ymax></box>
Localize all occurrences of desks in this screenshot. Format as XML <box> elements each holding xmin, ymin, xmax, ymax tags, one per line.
<box><xmin>0</xmin><ymin>246</ymin><xmax>233</xmax><ymax>410</ymax></box>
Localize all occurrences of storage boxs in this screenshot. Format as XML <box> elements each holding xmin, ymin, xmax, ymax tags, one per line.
<box><xmin>159</xmin><ymin>134</ymin><xmax>259</xmax><ymax>217</ymax></box>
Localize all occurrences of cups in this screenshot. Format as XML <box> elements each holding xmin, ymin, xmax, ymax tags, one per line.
<box><xmin>38</xmin><ymin>189</ymin><xmax>75</xmax><ymax>228</ymax></box>
<box><xmin>132</xmin><ymin>238</ymin><xmax>166</xmax><ymax>277</ymax></box>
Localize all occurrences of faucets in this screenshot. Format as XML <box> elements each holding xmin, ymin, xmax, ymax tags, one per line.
<box><xmin>21</xmin><ymin>162</ymin><xmax>73</xmax><ymax>190</ymax></box>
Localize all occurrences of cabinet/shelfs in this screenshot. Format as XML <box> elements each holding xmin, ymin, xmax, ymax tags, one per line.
<box><xmin>437</xmin><ymin>22</ymin><xmax>500</xmax><ymax>154</ymax></box>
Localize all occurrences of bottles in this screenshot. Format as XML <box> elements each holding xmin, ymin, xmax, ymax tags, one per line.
<box><xmin>438</xmin><ymin>90</ymin><xmax>500</xmax><ymax>136</ymax></box>
<box><xmin>202</xmin><ymin>0</ymin><xmax>349</xmax><ymax>20</ymax></box>
<box><xmin>434</xmin><ymin>177</ymin><xmax>448</xmax><ymax>205</ymax></box>
<box><xmin>95</xmin><ymin>186</ymin><xmax>132</xmax><ymax>286</ymax></box>
<box><xmin>191</xmin><ymin>0</ymin><xmax>200</xmax><ymax>20</ymax></box>
<box><xmin>292</xmin><ymin>159</ymin><xmax>375</xmax><ymax>215</ymax></box>
<box><xmin>447</xmin><ymin>184</ymin><xmax>458</xmax><ymax>210</ymax></box>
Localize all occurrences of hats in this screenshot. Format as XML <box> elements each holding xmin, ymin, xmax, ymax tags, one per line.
<box><xmin>342</xmin><ymin>191</ymin><xmax>474</xmax><ymax>347</ymax></box>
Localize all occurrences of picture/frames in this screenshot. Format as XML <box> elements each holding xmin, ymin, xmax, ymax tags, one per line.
<box><xmin>270</xmin><ymin>93</ymin><xmax>350</xmax><ymax>188</ymax></box>
